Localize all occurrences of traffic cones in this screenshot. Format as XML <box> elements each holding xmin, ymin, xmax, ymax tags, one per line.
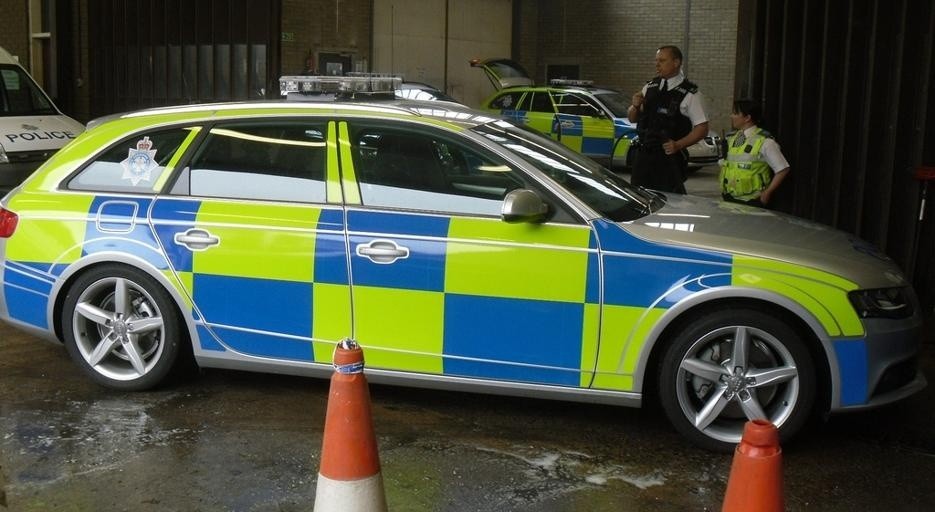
<box><xmin>711</xmin><ymin>417</ymin><xmax>789</xmax><ymax>511</ymax></box>
<box><xmin>304</xmin><ymin>338</ymin><xmax>395</xmax><ymax>509</ymax></box>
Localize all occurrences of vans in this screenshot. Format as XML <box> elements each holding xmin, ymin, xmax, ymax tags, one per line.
<box><xmin>0</xmin><ymin>43</ymin><xmax>87</xmax><ymax>197</ymax></box>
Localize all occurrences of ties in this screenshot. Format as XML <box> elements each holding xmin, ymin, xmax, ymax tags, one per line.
<box><xmin>661</xmin><ymin>80</ymin><xmax>666</xmax><ymax>94</ymax></box>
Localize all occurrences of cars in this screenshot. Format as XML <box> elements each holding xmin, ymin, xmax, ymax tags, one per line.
<box><xmin>467</xmin><ymin>53</ymin><xmax>726</xmax><ymax>180</ymax></box>
<box><xmin>350</xmin><ymin>69</ymin><xmax>460</xmax><ymax>108</ymax></box>
<box><xmin>0</xmin><ymin>67</ymin><xmax>929</xmax><ymax>459</ymax></box>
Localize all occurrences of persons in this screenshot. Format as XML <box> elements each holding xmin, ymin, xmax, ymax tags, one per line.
<box><xmin>716</xmin><ymin>98</ymin><xmax>791</xmax><ymax>209</ymax></box>
<box><xmin>627</xmin><ymin>45</ymin><xmax>710</xmax><ymax>195</ymax></box>
